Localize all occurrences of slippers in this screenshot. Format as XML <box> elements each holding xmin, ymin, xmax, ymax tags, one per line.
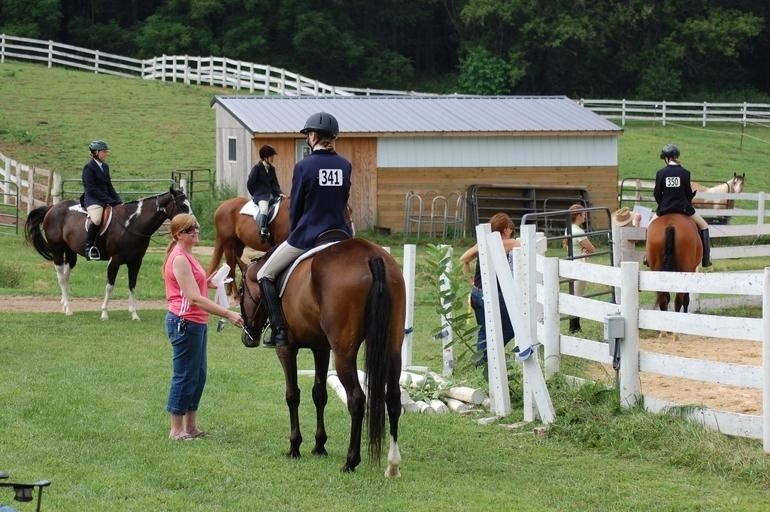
<box><xmin>167</xmin><ymin>430</ymin><xmax>212</xmax><ymax>442</ymax></box>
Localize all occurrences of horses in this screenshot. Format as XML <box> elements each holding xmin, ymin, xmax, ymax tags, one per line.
<box><xmin>24</xmin><ymin>184</ymin><xmax>200</xmax><ymax>321</ymax></box>
<box><xmin>645</xmin><ymin>189</ymin><xmax>704</xmax><ymax>340</ymax></box>
<box><xmin>207</xmin><ymin>197</ymin><xmax>355</xmax><ymax>334</ymax></box>
<box><xmin>693</xmin><ymin>171</ymin><xmax>745</xmax><ymax>220</ymax></box>
<box><xmin>235</xmin><ymin>235</ymin><xmax>406</xmax><ymax>478</ymax></box>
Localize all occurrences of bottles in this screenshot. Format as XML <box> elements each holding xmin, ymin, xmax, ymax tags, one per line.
<box><xmin>210</xmin><ymin>263</ymin><xmax>231</xmax><ymax>287</ymax></box>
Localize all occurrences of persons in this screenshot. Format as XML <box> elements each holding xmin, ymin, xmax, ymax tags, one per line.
<box><xmin>611</xmin><ymin>208</ymin><xmax>640</xmax><ymax>243</ymax></box>
<box><xmin>563</xmin><ymin>204</ymin><xmax>596</xmax><ymax>329</ymax></box>
<box><xmin>506</xmin><ymin>221</ymin><xmax>514</xmax><ymax>271</ymax></box>
<box><xmin>257</xmin><ymin>112</ymin><xmax>352</xmax><ymax>346</ymax></box>
<box><xmin>644</xmin><ymin>143</ymin><xmax>712</xmax><ymax>268</ymax></box>
<box><xmin>247</xmin><ymin>146</ymin><xmax>281</xmax><ymax>238</ymax></box>
<box><xmin>462</xmin><ymin>214</ymin><xmax>518</xmax><ymax>367</ymax></box>
<box><xmin>163</xmin><ymin>212</ymin><xmax>244</xmax><ymax>439</ymax></box>
<box><xmin>80</xmin><ymin>140</ymin><xmax>121</xmax><ymax>257</ymax></box>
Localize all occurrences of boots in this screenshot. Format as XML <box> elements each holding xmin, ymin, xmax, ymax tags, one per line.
<box><xmin>259</xmin><ymin>213</ymin><xmax>271</xmax><ymax>238</ymax></box>
<box><xmin>699</xmin><ymin>228</ymin><xmax>713</xmax><ymax>268</ymax></box>
<box><xmin>83</xmin><ymin>220</ymin><xmax>100</xmax><ymax>261</ymax></box>
<box><xmin>258</xmin><ymin>277</ymin><xmax>291</xmax><ymax>348</ymax></box>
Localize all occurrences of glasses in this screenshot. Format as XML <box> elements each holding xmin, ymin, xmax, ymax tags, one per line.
<box><xmin>181</xmin><ymin>225</ymin><xmax>199</xmax><ymax>234</ymax></box>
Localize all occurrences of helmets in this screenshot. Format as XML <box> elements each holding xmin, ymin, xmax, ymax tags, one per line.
<box><xmin>299</xmin><ymin>111</ymin><xmax>340</xmax><ymax>136</ymax></box>
<box><xmin>660</xmin><ymin>144</ymin><xmax>680</xmax><ymax>158</ymax></box>
<box><xmin>89</xmin><ymin>140</ymin><xmax>109</xmax><ymax>152</ymax></box>
<box><xmin>259</xmin><ymin>144</ymin><xmax>278</xmax><ymax>158</ymax></box>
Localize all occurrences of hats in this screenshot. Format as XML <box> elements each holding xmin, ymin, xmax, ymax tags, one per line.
<box><xmin>609</xmin><ymin>206</ymin><xmax>635</xmax><ymax>227</ymax></box>
